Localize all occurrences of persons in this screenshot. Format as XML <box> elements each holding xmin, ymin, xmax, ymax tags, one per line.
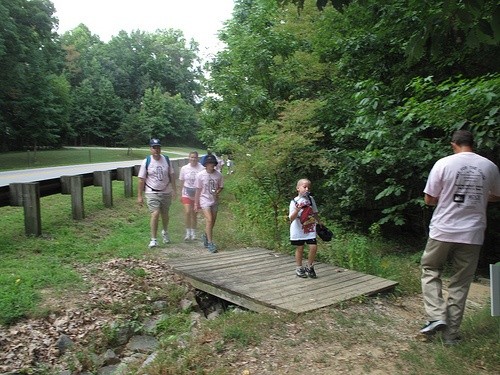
<box><xmin>202</xmin><ymin>148</ymin><xmax>225</xmax><ymax>172</ymax></box>
<box><xmin>137</xmin><ymin>138</ymin><xmax>177</xmax><ymax>248</ymax></box>
<box><xmin>289</xmin><ymin>178</ymin><xmax>323</xmax><ymax>279</ymax></box>
<box><xmin>178</xmin><ymin>152</ymin><xmax>206</xmax><ymax>241</ymax></box>
<box><xmin>193</xmin><ymin>155</ymin><xmax>224</xmax><ymax>253</ymax></box>
<box><xmin>419</xmin><ymin>129</ymin><xmax>500</xmax><ymax>344</ymax></box>
<box><xmin>225</xmin><ymin>149</ymin><xmax>234</xmax><ymax>174</ymax></box>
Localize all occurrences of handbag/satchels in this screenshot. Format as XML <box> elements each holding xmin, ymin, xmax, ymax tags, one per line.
<box><xmin>316</xmin><ymin>224</ymin><xmax>333</xmax><ymax>241</ymax></box>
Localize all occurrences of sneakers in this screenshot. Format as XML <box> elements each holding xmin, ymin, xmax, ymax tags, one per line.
<box><xmin>419</xmin><ymin>320</ymin><xmax>448</xmax><ymax>336</ymax></box>
<box><xmin>305</xmin><ymin>265</ymin><xmax>316</xmax><ymax>279</ymax></box>
<box><xmin>184</xmin><ymin>232</ymin><xmax>191</xmax><ymax>241</ymax></box>
<box><xmin>444</xmin><ymin>336</ymin><xmax>462</xmax><ymax>345</ymax></box>
<box><xmin>295</xmin><ymin>265</ymin><xmax>307</xmax><ymax>278</ymax></box>
<box><xmin>202</xmin><ymin>234</ymin><xmax>208</xmax><ymax>246</ymax></box>
<box><xmin>207</xmin><ymin>242</ymin><xmax>217</xmax><ymax>253</ymax></box>
<box><xmin>191</xmin><ymin>232</ymin><xmax>197</xmax><ymax>240</ymax></box>
<box><xmin>148</xmin><ymin>239</ymin><xmax>157</xmax><ymax>247</ymax></box>
<box><xmin>161</xmin><ymin>233</ymin><xmax>170</xmax><ymax>244</ymax></box>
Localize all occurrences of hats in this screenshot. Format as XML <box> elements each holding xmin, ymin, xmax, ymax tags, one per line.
<box><xmin>202</xmin><ymin>154</ymin><xmax>218</xmax><ymax>167</ymax></box>
<box><xmin>150</xmin><ymin>138</ymin><xmax>162</xmax><ymax>147</ymax></box>
